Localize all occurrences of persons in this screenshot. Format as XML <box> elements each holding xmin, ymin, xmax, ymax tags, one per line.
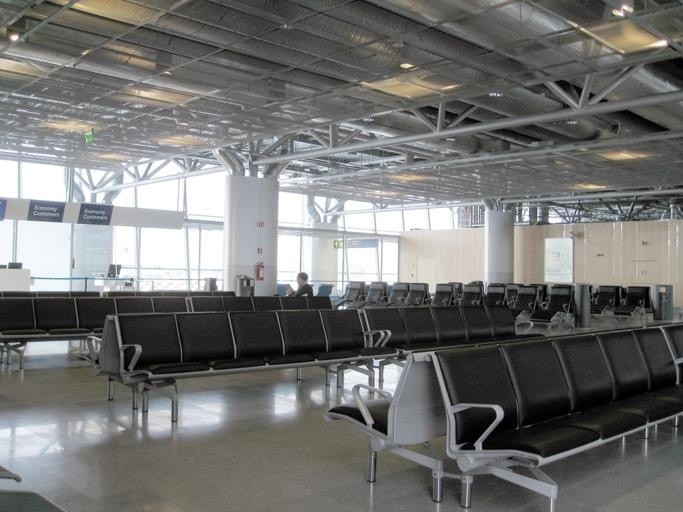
<box><xmin>286</xmin><ymin>272</ymin><xmax>314</xmax><ymax>297</ymax></box>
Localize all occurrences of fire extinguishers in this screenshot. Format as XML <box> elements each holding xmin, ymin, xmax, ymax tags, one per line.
<box><xmin>256</xmin><ymin>261</ymin><xmax>265</xmax><ymax>280</ymax></box>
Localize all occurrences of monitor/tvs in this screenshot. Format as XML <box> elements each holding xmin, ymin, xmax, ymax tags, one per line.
<box><xmin>0</xmin><ymin>265</ymin><xmax>7</xmax><ymax>269</ymax></box>
<box><xmin>107</xmin><ymin>264</ymin><xmax>122</xmax><ymax>277</ymax></box>
<box><xmin>8</xmin><ymin>262</ymin><xmax>23</xmax><ymax>269</ymax></box>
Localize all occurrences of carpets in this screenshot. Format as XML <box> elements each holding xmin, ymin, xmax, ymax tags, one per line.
<box><xmin>0</xmin><ymin>490</ymin><xmax>61</xmax><ymax>512</ymax></box>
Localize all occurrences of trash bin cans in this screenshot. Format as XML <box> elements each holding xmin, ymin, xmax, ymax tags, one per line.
<box><xmin>204</xmin><ymin>278</ymin><xmax>219</xmax><ymax>292</ymax></box>
<box><xmin>656</xmin><ymin>284</ymin><xmax>674</xmax><ymax>320</ymax></box>
<box><xmin>235</xmin><ymin>275</ymin><xmax>255</xmax><ymax>298</ymax></box>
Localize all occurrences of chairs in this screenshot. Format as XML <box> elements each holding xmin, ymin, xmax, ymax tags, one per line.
<box><xmin>323</xmin><ymin>321</ymin><xmax>681</xmax><ymax>512</ymax></box>
<box><xmin>0</xmin><ymin>280</ymin><xmax>682</xmax><ymax>422</ymax></box>
